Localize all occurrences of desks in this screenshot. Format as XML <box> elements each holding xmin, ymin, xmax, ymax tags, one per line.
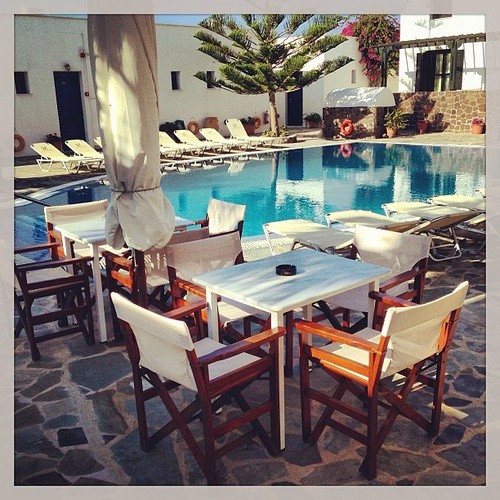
<box><xmin>54</xmin><ymin>215</ymin><xmax>197</xmax><ymax>346</ymax></box>
<box><xmin>193</xmin><ymin>248</ymin><xmax>390</xmax><ymax>453</ymax></box>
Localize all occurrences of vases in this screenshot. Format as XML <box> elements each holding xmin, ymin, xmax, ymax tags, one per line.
<box><xmin>418</xmin><ymin>121</ymin><xmax>428</xmax><ymax>134</ymax></box>
<box><xmin>52</xmin><ymin>142</ymin><xmax>61</xmax><ymax>150</ymax></box>
<box><xmin>472</xmin><ymin>125</ymin><xmax>484</xmax><ymax>133</ymax></box>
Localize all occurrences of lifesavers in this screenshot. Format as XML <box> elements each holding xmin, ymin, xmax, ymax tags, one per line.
<box><xmin>341</xmin><ymin>144</ymin><xmax>352</xmax><ymax>158</ymax></box>
<box><xmin>255</xmin><ymin>117</ymin><xmax>261</xmax><ymax>129</ymax></box>
<box><xmin>188</xmin><ymin>121</ymin><xmax>199</xmax><ymax>135</ymax></box>
<box><xmin>341</xmin><ymin>120</ymin><xmax>353</xmax><ymax>135</ymax></box>
<box><xmin>14</xmin><ymin>135</ymin><xmax>24</xmax><ymax>152</ymax></box>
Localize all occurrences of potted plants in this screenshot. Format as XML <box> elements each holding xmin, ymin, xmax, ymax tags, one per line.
<box><xmin>304</xmin><ymin>113</ymin><xmax>321</xmax><ymax>127</ymax></box>
<box><xmin>384</xmin><ymin>110</ymin><xmax>408</xmax><ymax>137</ymax></box>
<box><xmin>241</xmin><ymin>117</ymin><xmax>256</xmax><ymax>133</ymax></box>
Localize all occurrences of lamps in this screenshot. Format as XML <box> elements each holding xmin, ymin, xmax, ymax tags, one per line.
<box><xmin>65</xmin><ymin>64</ymin><xmax>70</xmax><ymax>72</ymax></box>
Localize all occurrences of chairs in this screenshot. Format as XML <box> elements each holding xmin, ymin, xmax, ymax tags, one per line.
<box><xmin>14</xmin><ymin>198</ymin><xmax>486</xmax><ymax>486</ymax></box>
<box><xmin>30</xmin><ymin>118</ymin><xmax>282</xmax><ymax>175</ymax></box>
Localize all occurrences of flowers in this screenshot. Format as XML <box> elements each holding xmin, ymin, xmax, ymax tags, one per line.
<box><xmin>472</xmin><ymin>118</ymin><xmax>482</xmax><ymax>125</ymax></box>
<box><xmin>46</xmin><ymin>133</ymin><xmax>62</xmax><ymax>142</ymax></box>
